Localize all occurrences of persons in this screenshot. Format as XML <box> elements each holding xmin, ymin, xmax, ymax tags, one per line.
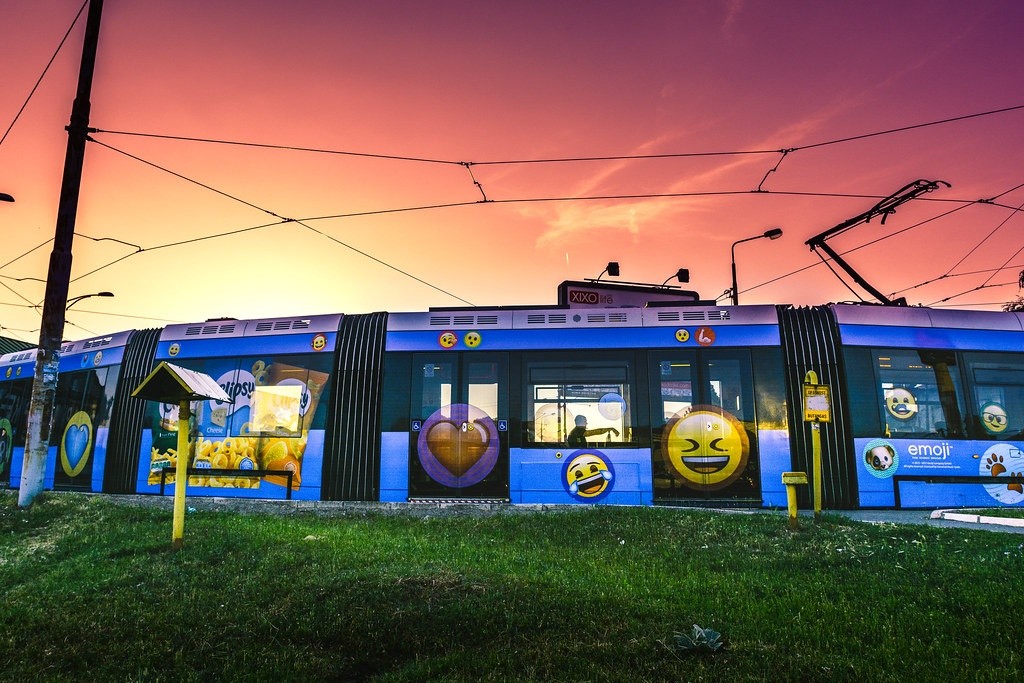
<box><xmin>566</xmin><ymin>414</ymin><xmax>618</xmax><ymax>447</ymax></box>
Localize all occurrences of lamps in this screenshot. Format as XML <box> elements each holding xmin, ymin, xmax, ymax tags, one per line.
<box><xmin>661</xmin><ymin>268</ymin><xmax>690</xmax><ymax>289</ymax></box>
<box><xmin>597</xmin><ymin>262</ymin><xmax>620</xmax><ymax>283</ymax></box>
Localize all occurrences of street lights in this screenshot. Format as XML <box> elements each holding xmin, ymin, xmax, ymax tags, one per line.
<box><xmin>730</xmin><ymin>227</ymin><xmax>783</xmax><ymax>305</ymax></box>
<box><xmin>16</xmin><ymin>292</ymin><xmax>114</xmax><ymax>509</ymax></box>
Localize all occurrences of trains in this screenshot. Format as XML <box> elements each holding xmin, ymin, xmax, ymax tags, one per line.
<box><xmin>1</xmin><ymin>174</ymin><xmax>1024</xmax><ymax>504</ymax></box>
